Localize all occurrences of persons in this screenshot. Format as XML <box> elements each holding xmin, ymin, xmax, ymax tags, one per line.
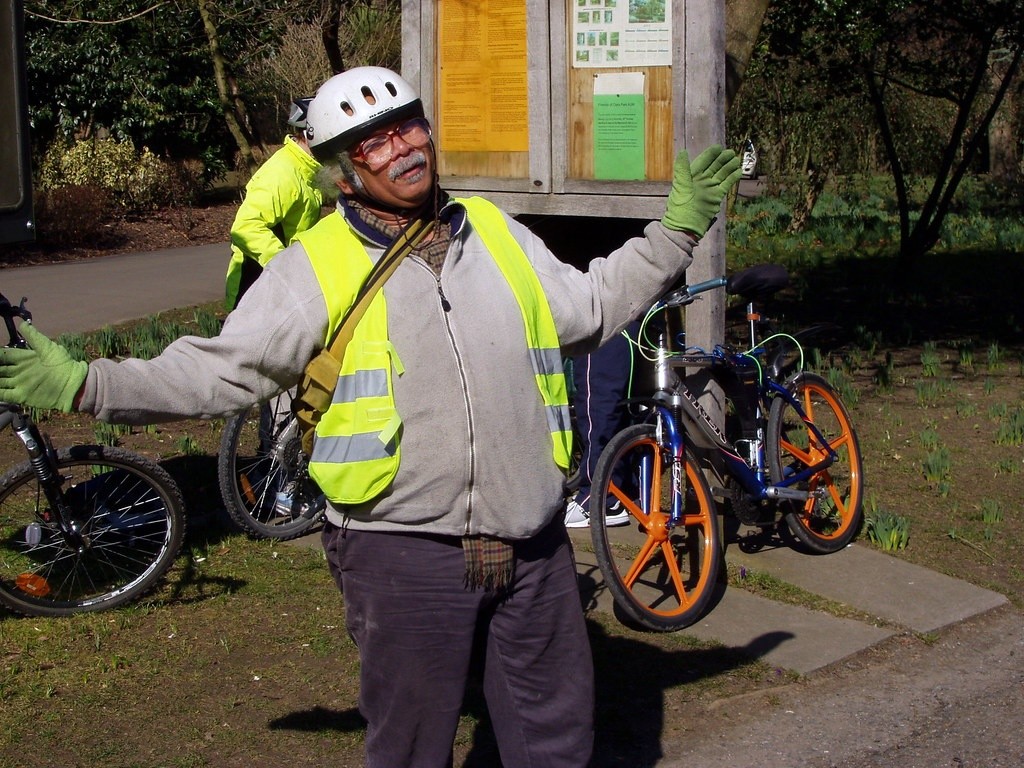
<box><xmin>0</xmin><ymin>66</ymin><xmax>741</xmax><ymax>767</ymax></box>
<box><xmin>560</xmin><ymin>320</ymin><xmax>647</xmax><ymax>529</ymax></box>
<box><xmin>225</xmin><ymin>95</ymin><xmax>325</xmax><ymax>522</ymax></box>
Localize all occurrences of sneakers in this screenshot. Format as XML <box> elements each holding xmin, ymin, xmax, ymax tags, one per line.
<box><xmin>275</xmin><ymin>491</ymin><xmax>326</xmax><ymax>522</ymax></box>
<box><xmin>563</xmin><ymin>497</ymin><xmax>632</xmax><ymax>530</ymax></box>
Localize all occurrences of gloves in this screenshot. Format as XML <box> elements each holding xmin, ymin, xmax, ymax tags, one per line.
<box><xmin>661</xmin><ymin>144</ymin><xmax>743</xmax><ymax>239</ymax></box>
<box><xmin>0</xmin><ymin>321</ymin><xmax>88</xmax><ymax>423</ymax></box>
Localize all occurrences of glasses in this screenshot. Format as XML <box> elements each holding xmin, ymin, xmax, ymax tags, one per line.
<box><xmin>350</xmin><ymin>115</ymin><xmax>432</xmax><ymax>172</ymax></box>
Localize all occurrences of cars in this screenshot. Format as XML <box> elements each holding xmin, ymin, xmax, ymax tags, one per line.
<box><xmin>741</xmin><ymin>139</ymin><xmax>758</xmax><ymax>180</ymax></box>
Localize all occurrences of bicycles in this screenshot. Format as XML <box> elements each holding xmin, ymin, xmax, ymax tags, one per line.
<box><xmin>218</xmin><ymin>388</ymin><xmax>327</xmax><ymax>542</ymax></box>
<box><xmin>1</xmin><ymin>295</ymin><xmax>188</xmax><ymax>618</ymax></box>
<box><xmin>588</xmin><ymin>274</ymin><xmax>865</xmax><ymax>632</ymax></box>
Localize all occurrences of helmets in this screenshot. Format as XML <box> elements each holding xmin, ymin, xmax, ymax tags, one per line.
<box><xmin>287</xmin><ymin>96</ymin><xmax>326</xmax><ymax>129</ymax></box>
<box><xmin>307</xmin><ymin>66</ymin><xmax>424</xmax><ymax>162</ymax></box>
